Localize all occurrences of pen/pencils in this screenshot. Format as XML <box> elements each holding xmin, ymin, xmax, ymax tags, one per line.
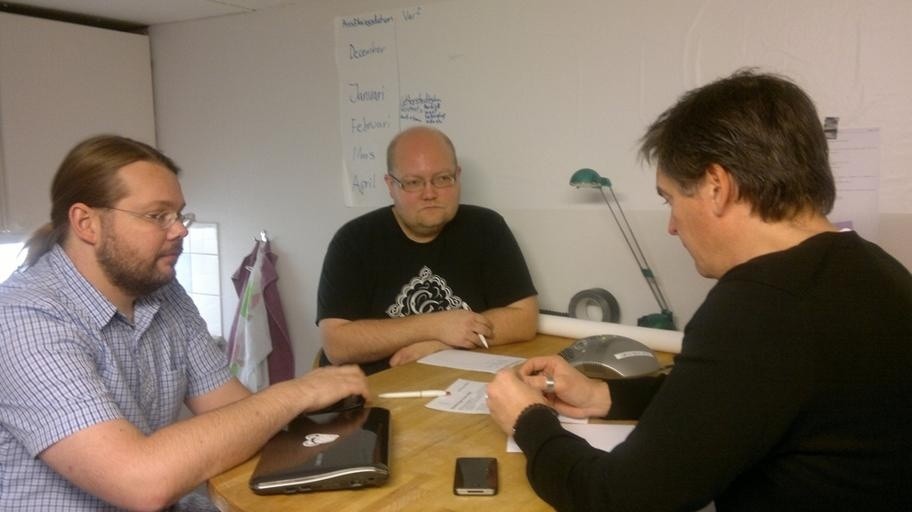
<box><xmin>460</xmin><ymin>301</ymin><xmax>489</xmax><ymax>350</ymax></box>
<box><xmin>377</xmin><ymin>390</ymin><xmax>450</xmax><ymax>399</ymax></box>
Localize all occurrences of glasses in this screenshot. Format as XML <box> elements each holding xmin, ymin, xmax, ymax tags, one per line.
<box><xmin>387</xmin><ymin>173</ymin><xmax>457</xmax><ymax>193</ymax></box>
<box><xmin>101</xmin><ymin>204</ymin><xmax>197</xmax><ymax>230</ymax></box>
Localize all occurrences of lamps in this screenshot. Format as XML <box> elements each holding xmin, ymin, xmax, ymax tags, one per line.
<box><xmin>568</xmin><ymin>168</ymin><xmax>675</xmax><ymax>333</ymax></box>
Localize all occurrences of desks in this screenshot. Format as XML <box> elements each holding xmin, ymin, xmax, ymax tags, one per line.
<box><xmin>202</xmin><ymin>334</ymin><xmax>688</xmax><ymax>511</ymax></box>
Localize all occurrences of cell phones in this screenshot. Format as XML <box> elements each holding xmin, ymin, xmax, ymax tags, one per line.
<box><xmin>452</xmin><ymin>456</ymin><xmax>499</xmax><ymax>496</ymax></box>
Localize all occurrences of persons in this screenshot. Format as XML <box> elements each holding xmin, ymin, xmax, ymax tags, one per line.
<box><xmin>483</xmin><ymin>72</ymin><xmax>912</xmax><ymax>511</ymax></box>
<box><xmin>1</xmin><ymin>134</ymin><xmax>372</xmax><ymax>512</ymax></box>
<box><xmin>316</xmin><ymin>126</ymin><xmax>538</xmax><ymax>376</ymax></box>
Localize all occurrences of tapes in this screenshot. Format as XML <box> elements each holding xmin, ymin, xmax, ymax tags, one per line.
<box><xmin>568</xmin><ymin>288</ymin><xmax>620</xmax><ymax>323</ymax></box>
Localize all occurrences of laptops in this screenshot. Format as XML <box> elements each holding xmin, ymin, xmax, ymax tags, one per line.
<box><xmin>249</xmin><ymin>407</ymin><xmax>391</xmax><ymax>496</ymax></box>
